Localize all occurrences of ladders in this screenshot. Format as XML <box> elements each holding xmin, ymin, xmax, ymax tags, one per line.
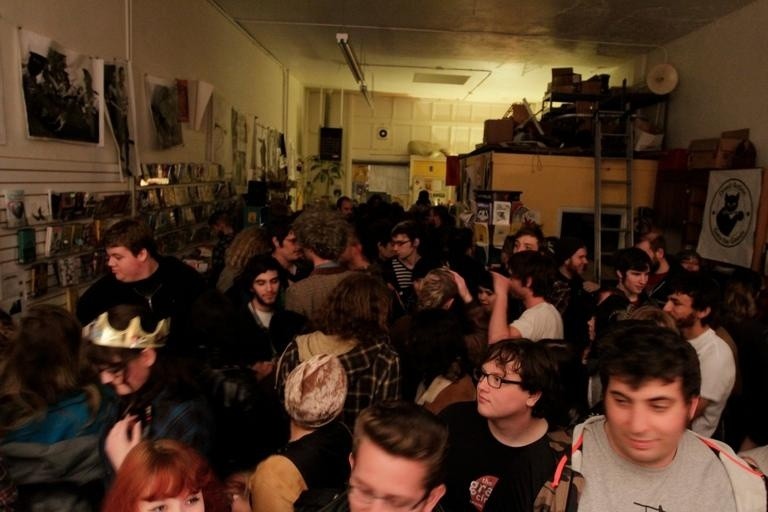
<box><xmin>594</xmin><ymin>101</ymin><xmax>633</xmax><ymax>284</ymax></box>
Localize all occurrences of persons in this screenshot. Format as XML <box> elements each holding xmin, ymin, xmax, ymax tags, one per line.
<box><xmin>106</xmin><ymin>66</ymin><xmax>134</xmax><ymax>177</ymax></box>
<box><xmin>21</xmin><ymin>52</ymin><xmax>95</xmax><ymax>144</ymax></box>
<box><xmin>0</xmin><ymin>183</ymin><xmax>768</xmax><ymax>512</ymax></box>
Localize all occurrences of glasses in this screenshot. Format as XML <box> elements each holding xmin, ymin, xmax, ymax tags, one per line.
<box><xmin>472</xmin><ymin>366</ymin><xmax>524</xmax><ymax>390</ymax></box>
<box><xmin>388</xmin><ymin>238</ymin><xmax>410</xmax><ymax>246</ymax></box>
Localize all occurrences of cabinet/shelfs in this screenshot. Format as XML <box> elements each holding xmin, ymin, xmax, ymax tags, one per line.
<box><xmin>6</xmin><ymin>178</ymin><xmax>235</xmax><ymax>318</ymax></box>
<box><xmin>408</xmin><ymin>154</ymin><xmax>456</xmax><ymax>214</ymax></box>
<box><xmin>654</xmin><ymin>171</ymin><xmax>707</xmax><ymax>245</ymax></box>
<box><xmin>542</xmin><ymin>92</ymin><xmax>671</xmax><ymax>159</ymax></box>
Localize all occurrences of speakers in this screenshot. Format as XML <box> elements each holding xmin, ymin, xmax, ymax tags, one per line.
<box><xmin>319</xmin><ymin>126</ymin><xmax>342</xmax><ymax>161</ymax></box>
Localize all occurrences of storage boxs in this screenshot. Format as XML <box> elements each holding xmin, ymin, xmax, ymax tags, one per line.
<box><xmin>483</xmin><ymin>119</ymin><xmax>513</xmax><ymax>142</ymax></box>
<box><xmin>690</xmin><ymin>128</ymin><xmax>749</xmax><ymax>168</ymax></box>
<box><xmin>633</xmin><ymin>118</ymin><xmax>664</xmax><ymax>152</ymax></box>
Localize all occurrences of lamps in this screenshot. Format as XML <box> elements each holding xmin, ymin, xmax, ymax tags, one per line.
<box><xmin>360</xmin><ymin>83</ymin><xmax>373</xmax><ymax>107</ymax></box>
<box><xmin>336</xmin><ymin>31</ymin><xmax>366</xmax><ymax>85</ymax></box>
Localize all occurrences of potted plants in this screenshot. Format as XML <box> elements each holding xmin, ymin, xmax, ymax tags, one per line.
<box><xmin>305</xmin><ymin>154</ymin><xmax>345</xmax><ymax>200</ymax></box>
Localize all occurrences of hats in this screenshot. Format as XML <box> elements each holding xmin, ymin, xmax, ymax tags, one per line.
<box><xmin>284</xmin><ymin>353</ymin><xmax>349</xmax><ymax>429</ymax></box>
